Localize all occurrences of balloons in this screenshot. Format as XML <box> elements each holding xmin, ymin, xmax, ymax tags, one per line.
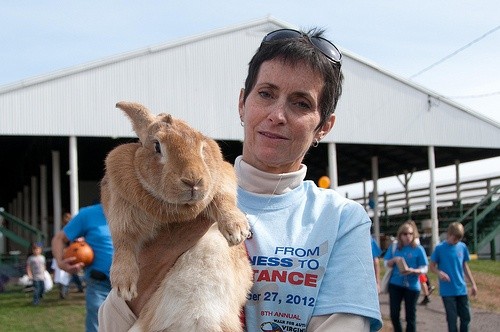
<box><xmin>318</xmin><ymin>175</ymin><xmax>330</xmax><ymax>189</ymax></box>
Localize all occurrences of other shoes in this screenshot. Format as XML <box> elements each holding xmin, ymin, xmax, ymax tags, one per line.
<box><xmin>419</xmin><ymin>297</ymin><xmax>431</xmax><ymax>305</ymax></box>
<box><xmin>428</xmin><ymin>285</ymin><xmax>435</xmax><ymax>294</ymax></box>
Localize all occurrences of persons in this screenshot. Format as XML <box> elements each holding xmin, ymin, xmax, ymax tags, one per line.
<box><xmin>430</xmin><ymin>222</ymin><xmax>477</xmax><ymax>332</ymax></box>
<box><xmin>98</xmin><ymin>24</ymin><xmax>383</xmax><ymax>332</ymax></box>
<box><xmin>24</xmin><ymin>216</ymin><xmax>84</xmax><ymax>307</ymax></box>
<box><xmin>52</xmin><ymin>204</ymin><xmax>114</xmax><ymax>332</ymax></box>
<box><xmin>370</xmin><ymin>219</ymin><xmax>434</xmax><ymax>332</ymax></box>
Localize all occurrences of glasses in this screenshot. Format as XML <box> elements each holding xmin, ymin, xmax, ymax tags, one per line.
<box><xmin>402</xmin><ymin>232</ymin><xmax>414</xmax><ymax>236</ymax></box>
<box><xmin>259</xmin><ymin>28</ymin><xmax>343</xmax><ymax>100</ymax></box>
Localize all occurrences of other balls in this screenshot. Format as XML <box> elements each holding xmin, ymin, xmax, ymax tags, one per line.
<box><xmin>64</xmin><ymin>242</ymin><xmax>94</xmax><ymax>266</ymax></box>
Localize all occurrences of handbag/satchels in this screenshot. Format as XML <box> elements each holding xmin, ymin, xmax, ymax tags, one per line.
<box><xmin>379</xmin><ymin>266</ymin><xmax>393</xmax><ymax>294</ymax></box>
